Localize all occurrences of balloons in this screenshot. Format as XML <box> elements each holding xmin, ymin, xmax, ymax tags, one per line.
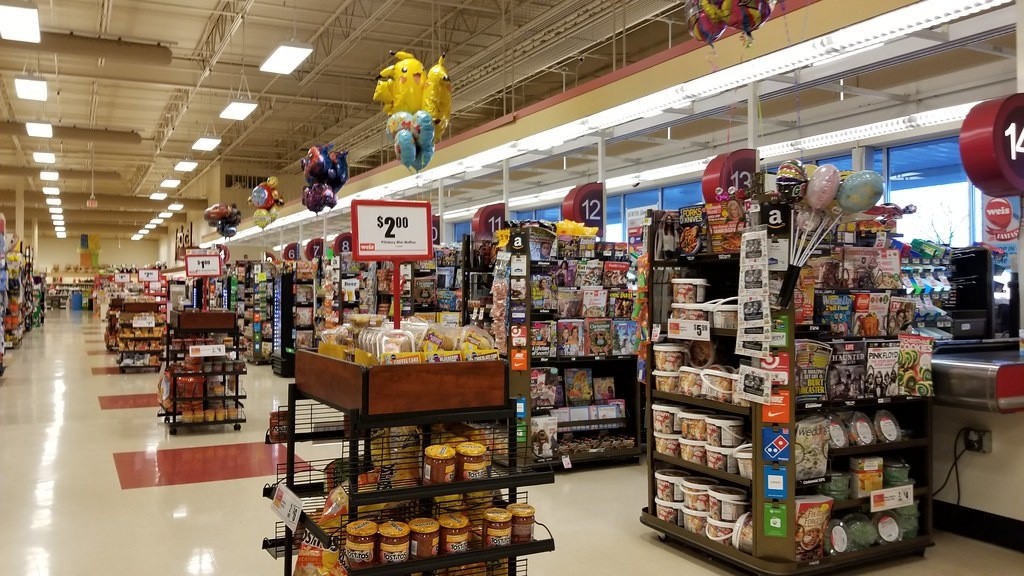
<box><xmin>300</xmin><ymin>142</ymin><xmax>349</xmax><ymax>216</ymax></box>
<box><xmin>247</xmin><ymin>176</ymin><xmax>284</xmax><ymax>230</ymax></box>
<box><xmin>372</xmin><ymin>51</ymin><xmax>451</xmax><ymax>174</ymax></box>
<box><xmin>684</xmin><ymin>1</ymin><xmax>775</xmax><ymax>50</ymax></box>
<box><xmin>204</xmin><ymin>203</ymin><xmax>242</xmax><ymax>238</ymax></box>
<box><xmin>778</xmin><ymin>158</ymin><xmax>884</xmax><ymax>215</ymax></box>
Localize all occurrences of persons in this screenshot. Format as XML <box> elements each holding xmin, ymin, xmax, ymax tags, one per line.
<box><xmin>536</xmin><ymin>277</ymin><xmax>551</xmax><ymax>308</ymax></box>
<box><xmin>661</xmin><ymin>215</ymin><xmax>679</xmax><ymax>257</ymax></box>
<box><xmin>538</xmin><ymin>242</ymin><xmax>552</xmax><ymax>261</ymax></box>
<box><xmin>866</xmin><ymin>371</ymin><xmax>898</xmax><ymax>395</ymax></box>
<box><xmin>554</xmin><ymin>261</ymin><xmax>571</xmax><ymax>287</ymax></box>
<box><xmin>890</xmin><ymin>302</ymin><xmax>915</xmax><ymax>334</ymax></box>
<box><xmin>561</xmin><ymin>325</ymin><xmax>570</xmax><ymax>351</ymax></box>
<box><xmin>571</xmin><ymin>325</ymin><xmax>581</xmax><ymax>353</ymax></box>
<box><xmin>566</xmin><ymin>234</ymin><xmax>579</xmax><ymax>254</ymax></box>
<box><xmin>533</xmin><ymin>326</ymin><xmax>551</xmax><ymax>356</ymax></box>
<box><xmin>723</xmin><ymin>201</ymin><xmax>745</xmax><ymax>224</ymax></box>
<box><xmin>742</xmin><ymin>237</ymin><xmax>765</xmax><ymax>399</ymax></box>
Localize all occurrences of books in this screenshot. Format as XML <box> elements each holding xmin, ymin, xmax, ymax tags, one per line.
<box><xmin>889</xmin><ymin>296</ymin><xmax>917</xmax><ymax>336</ymax></box>
<box><xmin>528</xmin><ymin>224</ymin><xmax>640</xmax><ymax>457</ymax></box>
<box><xmin>795</xmin><ymin>339</ymin><xmax>866</xmax><ymax>403</ymax></box>
<box><xmin>898</xmin><ymin>335</ymin><xmax>936</xmax><ymax>398</ymax></box>
<box><xmin>794</xmin><ymin>248</ymin><xmax>902</xmax><ymax>338</ymax></box>
<box><xmin>865</xmin><ymin>339</ymin><xmax>900</xmax><ymax>398</ymax></box>
<box><xmin>652</xmin><ymin>200</ymin><xmax>744</xmax><ymax>259</ymax></box>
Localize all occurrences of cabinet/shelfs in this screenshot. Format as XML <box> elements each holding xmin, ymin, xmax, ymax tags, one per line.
<box><xmin>641</xmin><ymin>207</ymin><xmax>937</xmax><ymax>576</ymax></box>
<box><xmin>193</xmin><ymin>240</ymin><xmax>463</xmax><ymax>375</ymax></box>
<box><xmin>101</xmin><ymin>292</ymin><xmax>169</xmax><ymax>375</ymax></box>
<box><xmin>261</xmin><ymin>345</ymin><xmax>555</xmax><ymax>576</ymax></box>
<box><xmin>455</xmin><ymin>235</ymin><xmax>648</xmax><ymax>474</ymax></box>
<box><xmin>0</xmin><ymin>246</ymin><xmax>46</xmax><ymax>376</ymax></box>
<box><xmin>47</xmin><ymin>283</ymin><xmax>94</xmax><ymax>306</ymax></box>
<box><xmin>158</xmin><ymin>308</ymin><xmax>247</xmax><ymax>434</ymax></box>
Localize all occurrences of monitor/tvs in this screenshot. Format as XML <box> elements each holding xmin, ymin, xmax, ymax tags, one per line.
<box><xmin>950</xmin><ymin>247</ymin><xmax>995</xmax><ymax>340</ymax></box>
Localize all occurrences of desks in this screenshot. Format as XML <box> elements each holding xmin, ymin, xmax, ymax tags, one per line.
<box><xmin>50</xmin><ymin>296</ymin><xmax>68</xmax><ymax>308</ymax></box>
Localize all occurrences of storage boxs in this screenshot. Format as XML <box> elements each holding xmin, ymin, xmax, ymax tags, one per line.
<box><xmin>0</xmin><ymin>213</ymin><xmax>9</xmax><ymax>292</ymax></box>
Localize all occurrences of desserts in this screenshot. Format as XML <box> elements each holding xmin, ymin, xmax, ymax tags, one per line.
<box><xmin>898</xmin><ymin>349</ymin><xmax>933</xmax><ymax>397</ymax></box>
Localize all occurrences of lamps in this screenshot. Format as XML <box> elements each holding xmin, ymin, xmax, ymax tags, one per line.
<box><xmin>130</xmin><ymin>102</ymin><xmax>198</xmax><ymax>242</ymax></box>
<box><xmin>220</xmin><ymin>19</ymin><xmax>258</xmax><ymax>123</ymax></box>
<box><xmin>258</xmin><ymin>2</ymin><xmax>313</xmax><ymax>76</ymax></box>
<box><xmin>191</xmin><ymin>72</ymin><xmax>221</xmax><ymax>152</ymax></box>
<box><xmin>0</xmin><ymin>0</ymin><xmax>43</xmax><ymax>44</ymax></box>
<box><xmin>15</xmin><ymin>41</ymin><xmax>67</xmax><ymax>243</ymax></box>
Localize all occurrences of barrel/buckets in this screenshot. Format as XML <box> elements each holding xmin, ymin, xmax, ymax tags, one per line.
<box><xmin>652</xmin><ymin>279</ymin><xmax>753</xmax><ymax>555</ymax></box>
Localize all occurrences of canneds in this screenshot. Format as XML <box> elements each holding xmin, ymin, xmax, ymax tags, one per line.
<box><xmin>346</xmin><ymin>443</ymin><xmax>535</xmax><ymax>570</ymax></box>
<box><xmin>172</xmin><ymin>337</ymin><xmax>244</xmax><ymax>423</ymax></box>
